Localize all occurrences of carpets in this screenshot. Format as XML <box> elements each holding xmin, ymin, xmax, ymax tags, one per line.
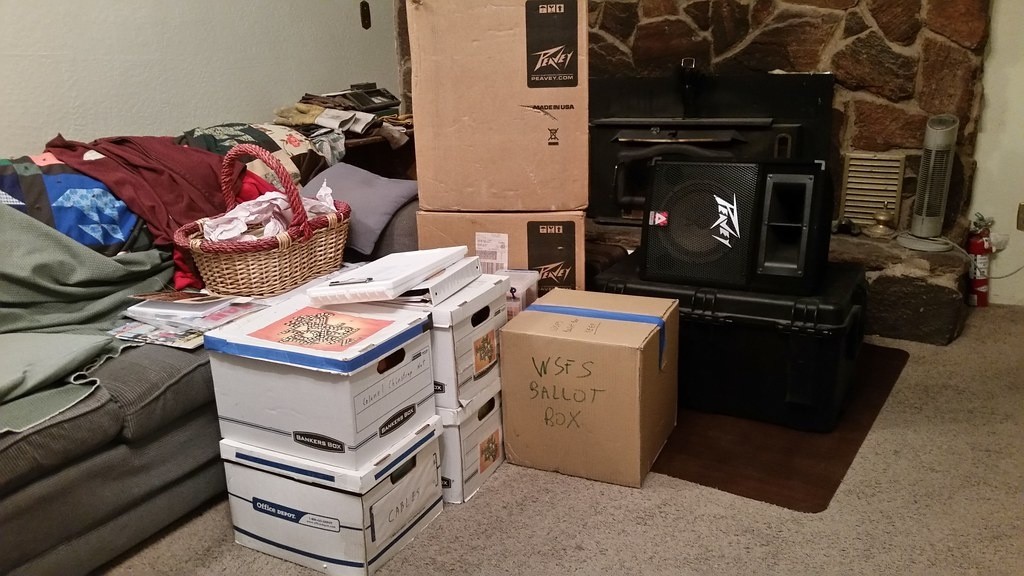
<box><xmin>645</xmin><ymin>339</ymin><xmax>907</xmax><ymax>516</ymax></box>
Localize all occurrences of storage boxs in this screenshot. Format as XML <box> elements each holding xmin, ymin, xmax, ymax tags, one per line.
<box><xmin>172</xmin><ymin>143</ymin><xmax>352</xmax><ymax>293</ymax></box>
<box><xmin>208</xmin><ymin>1</ymin><xmax>684</xmax><ymax>574</ymax></box>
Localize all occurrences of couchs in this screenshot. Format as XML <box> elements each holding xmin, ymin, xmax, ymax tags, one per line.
<box><xmin>0</xmin><ymin>109</ymin><xmax>418</xmax><ymax>575</ymax></box>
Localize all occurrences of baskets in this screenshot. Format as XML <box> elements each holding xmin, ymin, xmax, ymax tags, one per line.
<box><xmin>169</xmin><ymin>142</ymin><xmax>350</xmax><ymax>300</ymax></box>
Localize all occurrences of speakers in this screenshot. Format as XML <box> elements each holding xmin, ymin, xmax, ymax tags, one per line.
<box><xmin>639</xmin><ymin>156</ymin><xmax>836</xmax><ymax>295</ymax></box>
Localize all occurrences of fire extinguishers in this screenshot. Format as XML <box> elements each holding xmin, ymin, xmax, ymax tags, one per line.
<box><xmin>966</xmin><ymin>212</ymin><xmax>997</xmax><ymax>307</ymax></box>
